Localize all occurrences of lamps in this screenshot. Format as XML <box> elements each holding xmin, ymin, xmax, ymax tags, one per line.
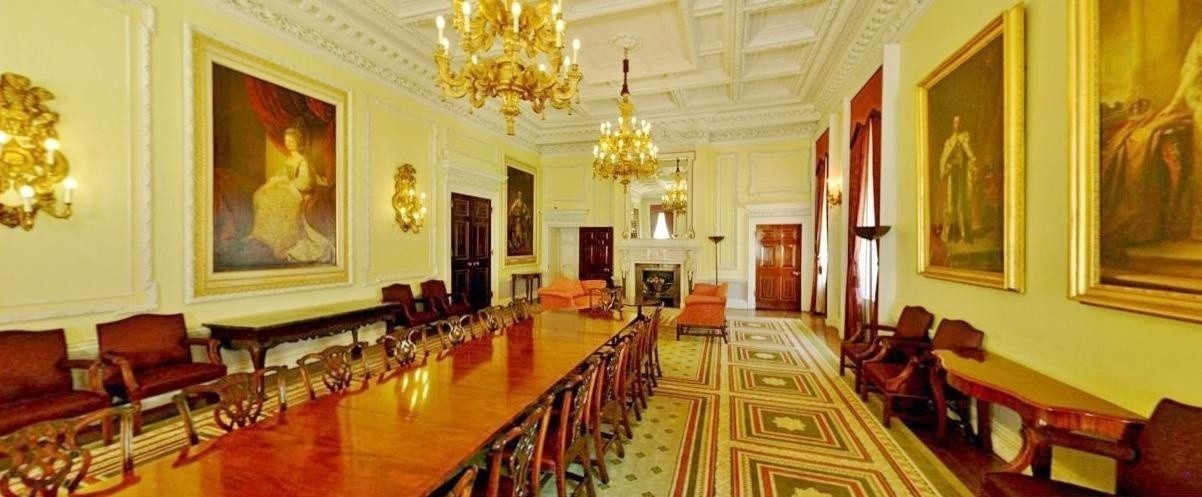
<box><xmin>660</xmin><ymin>156</ymin><xmax>689</xmax><ymax>219</ymax></box>
<box><xmin>851</xmin><ymin>225</ymin><xmax>893</xmax><ymax>322</ymax></box>
<box><xmin>590</xmin><ymin>41</ymin><xmax>659</xmax><ymax>191</ymax></box>
<box><xmin>390</xmin><ymin>161</ymin><xmax>429</xmax><ymax>236</ymax></box>
<box><xmin>708</xmin><ymin>235</ymin><xmax>724</xmax><ymax>284</ymax></box>
<box><xmin>825</xmin><ymin>175</ymin><xmax>843</xmax><ymax>209</ymax></box>
<box><xmin>427</xmin><ymin>0</ymin><xmax>586</xmax><ymax>142</ymax></box>
<box><xmin>0</xmin><ymin>71</ymin><xmax>80</xmax><ymax>236</ymax></box>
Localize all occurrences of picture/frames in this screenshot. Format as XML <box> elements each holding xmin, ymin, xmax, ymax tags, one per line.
<box><xmin>180</xmin><ymin>12</ymin><xmax>356</xmax><ymax>307</ymax></box>
<box><xmin>911</xmin><ymin>1</ymin><xmax>1032</xmax><ymax>296</ymax></box>
<box><xmin>1061</xmin><ymin>1</ymin><xmax>1202</xmax><ymax>324</ymax></box>
<box><xmin>502</xmin><ymin>152</ymin><xmax>541</xmax><ymax>273</ymax></box>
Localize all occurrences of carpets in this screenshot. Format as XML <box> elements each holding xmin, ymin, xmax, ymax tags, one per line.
<box><xmin>1</xmin><ymin>306</ymin><xmax>976</xmax><ymax>497</ymax></box>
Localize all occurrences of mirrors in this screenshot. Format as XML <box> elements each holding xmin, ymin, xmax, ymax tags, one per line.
<box><xmin>623</xmin><ymin>149</ymin><xmax>696</xmax><ymax>241</ymax></box>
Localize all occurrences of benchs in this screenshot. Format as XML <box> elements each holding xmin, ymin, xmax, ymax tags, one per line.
<box><xmin>675</xmin><ymin>283</ymin><xmax>730</xmax><ymax>344</ymax></box>
<box><xmin>539</xmin><ymin>277</ymin><xmax>606</xmax><ymax>308</ymax></box>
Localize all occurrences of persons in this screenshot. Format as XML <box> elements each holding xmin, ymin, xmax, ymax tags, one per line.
<box><xmin>938</xmin><ymin>116</ymin><xmax>981</xmax><ymax>244</ymax></box>
<box><xmin>250</xmin><ymin>126</ymin><xmax>337</xmax><ymax>264</ymax></box>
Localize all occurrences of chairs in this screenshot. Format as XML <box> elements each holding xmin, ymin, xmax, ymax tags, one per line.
<box><xmin>601</xmin><ymin>305</ymin><xmax>662</xmax><ymax>440</ymax></box>
<box><xmin>835</xmin><ymin>303</ymin><xmax>939</xmax><ymax>397</ymax></box>
<box><xmin>486</xmin><ymin>358</ymin><xmax>601</xmax><ymax>496</ymax></box>
<box><xmin>551</xmin><ymin>338</ymin><xmax>633</xmax><ymax>484</ymax></box>
<box><xmin>1</xmin><ymin>327</ymin><xmax>110</xmax><ymax>459</ymax></box>
<box><xmin>428</xmin><ymin>394</ymin><xmax>555</xmax><ymax>495</ymax></box>
<box><xmin>96</xmin><ymin>312</ymin><xmax>229</xmax><ymax>437</ymax></box>
<box><xmin>448</xmin><ymin>464</ymin><xmax>478</xmax><ymax>495</ymax></box>
<box><xmin>382</xmin><ymin>283</ymin><xmax>441</xmax><ymax>333</ymax></box>
<box><xmin>421</xmin><ymin>279</ymin><xmax>470</xmax><ymax>316</ymax></box>
<box><xmin>985</xmin><ymin>396</ymin><xmax>1202</xmax><ymax>497</ymax></box>
<box><xmin>588</xmin><ymin>287</ymin><xmax>625</xmax><ymax>308</ymax></box>
<box><xmin>858</xmin><ymin>318</ymin><xmax>989</xmax><ymax>438</ymax></box>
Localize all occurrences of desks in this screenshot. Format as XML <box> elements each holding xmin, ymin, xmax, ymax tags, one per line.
<box><xmin>926</xmin><ymin>344</ymin><xmax>1151</xmax><ymax>480</ymax></box>
<box><xmin>202</xmin><ymin>302</ymin><xmax>400</xmax><ymax>400</ymax></box>
<box><xmin>58</xmin><ymin>309</ymin><xmax>639</xmax><ymax>496</ymax></box>
